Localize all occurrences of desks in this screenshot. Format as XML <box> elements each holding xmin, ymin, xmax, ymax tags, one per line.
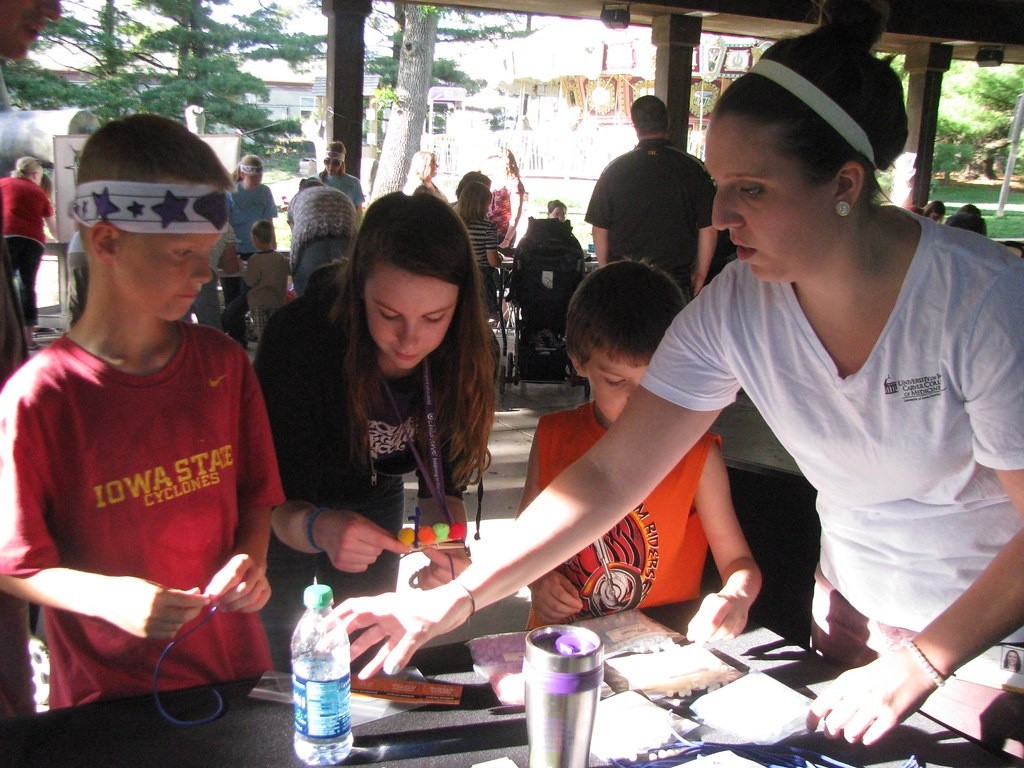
<box><xmin>0</xmin><ymin>598</ymin><xmax>1023</xmax><ymax>768</ymax></box>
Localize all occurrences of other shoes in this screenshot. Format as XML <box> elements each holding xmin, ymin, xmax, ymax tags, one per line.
<box><xmin>26</xmin><ymin>340</ymin><xmax>40</xmax><ymax>349</ymax></box>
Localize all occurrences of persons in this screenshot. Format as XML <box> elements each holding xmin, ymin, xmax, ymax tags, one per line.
<box><xmin>0</xmin><ymin>0</ymin><xmax>62</xmax><ymax>63</ymax></box>
<box><xmin>312</xmin><ymin>141</ymin><xmax>365</xmax><ymax>218</ymax></box>
<box><xmin>67</xmin><ymin>231</ymin><xmax>88</xmax><ymax>332</ymax></box>
<box><xmin>583</xmin><ymin>96</ymin><xmax>718</xmax><ymax>302</ymax></box>
<box><xmin>1004</xmin><ymin>651</ymin><xmax>1021</xmax><ymax>673</ymax></box>
<box><xmin>450</xmin><ymin>147</ymin><xmax>566</xmax><ymax>322</ymax></box>
<box><xmin>515</xmin><ymin>261</ymin><xmax>763</xmax><ymax>646</ymax></box>
<box><xmin>0</xmin><ymin>115</ymin><xmax>283</xmax><ymax>712</ymax></box>
<box><xmin>401</xmin><ymin>152</ymin><xmax>447</xmax><ymax>200</ymax></box>
<box><xmin>314</xmin><ymin>1</ymin><xmax>1024</xmax><ymax>768</ymax></box>
<box><xmin>0</xmin><ymin>155</ymin><xmax>58</xmax><ymax>349</ymax></box>
<box><xmin>901</xmin><ymin>201</ymin><xmax>945</xmax><ymax>222</ymax></box>
<box><xmin>945</xmin><ymin>204</ymin><xmax>987</xmax><ymax>235</ymax></box>
<box><xmin>181</xmin><ymin>154</ymin><xmax>356</xmax><ymax>346</ymax></box>
<box><xmin>250</xmin><ymin>192</ymin><xmax>495</xmax><ymax>670</ymax></box>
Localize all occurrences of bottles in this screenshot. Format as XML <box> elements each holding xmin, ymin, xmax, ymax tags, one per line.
<box><xmin>290</xmin><ymin>585</ymin><xmax>354</xmax><ymax>765</ymax></box>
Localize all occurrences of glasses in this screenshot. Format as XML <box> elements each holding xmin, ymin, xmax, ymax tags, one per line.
<box><xmin>324</xmin><ymin>159</ymin><xmax>340</xmax><ymax>167</ymax></box>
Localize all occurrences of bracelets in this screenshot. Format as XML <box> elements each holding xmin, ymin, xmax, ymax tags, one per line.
<box><xmin>904</xmin><ymin>641</ymin><xmax>946</xmax><ymax>688</ymax></box>
<box><xmin>307</xmin><ymin>509</ymin><xmax>324</xmax><ymax>551</ymax></box>
<box><xmin>452</xmin><ymin>580</ymin><xmax>475</xmax><ymax>615</ymax></box>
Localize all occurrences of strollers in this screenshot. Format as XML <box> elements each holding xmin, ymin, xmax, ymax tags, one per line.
<box><xmin>493</xmin><ymin>216</ymin><xmax>594</xmax><ymax>399</ymax></box>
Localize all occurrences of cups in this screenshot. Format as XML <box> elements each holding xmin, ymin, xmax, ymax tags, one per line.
<box><xmin>522</xmin><ymin>624</ymin><xmax>604</xmax><ymax>768</ymax></box>
